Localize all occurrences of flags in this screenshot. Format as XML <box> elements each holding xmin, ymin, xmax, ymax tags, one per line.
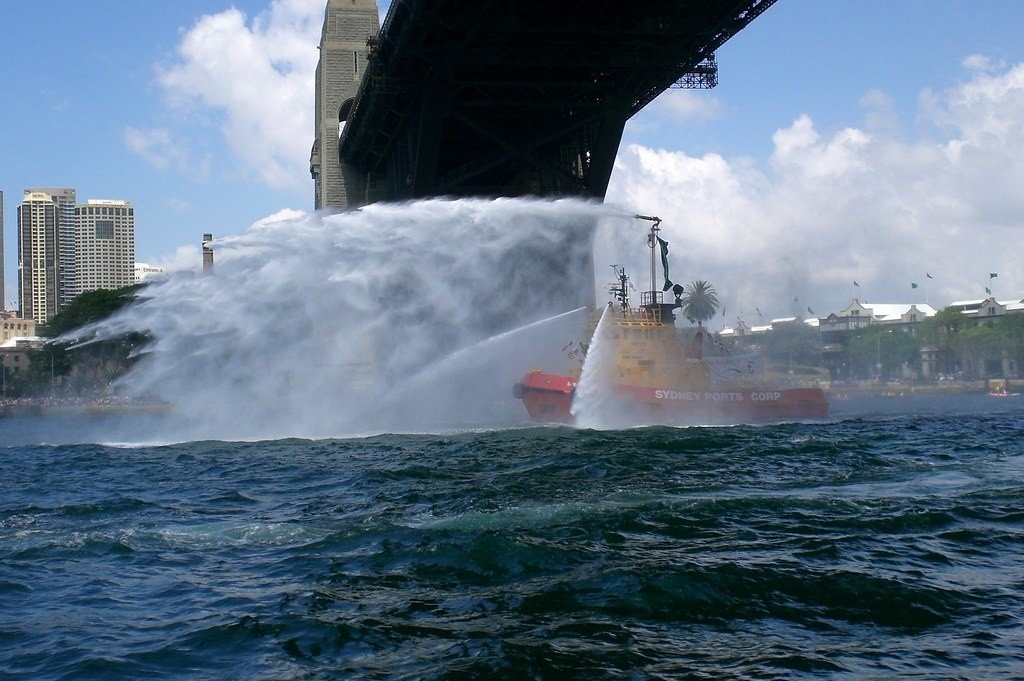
<box><xmin>722</xmin><ymin>272</ymin><xmax>998</xmax><ymax>317</ymax></box>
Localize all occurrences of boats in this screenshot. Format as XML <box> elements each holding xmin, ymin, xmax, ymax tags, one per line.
<box><xmin>512</xmin><ymin>215</ymin><xmax>832</xmax><ymax>428</ymax></box>
<box><xmin>988</xmin><ymin>392</ymin><xmax>1009</xmax><ymax>399</ymax></box>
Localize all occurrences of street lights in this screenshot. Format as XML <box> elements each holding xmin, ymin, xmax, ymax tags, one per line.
<box><xmin>3</xmin><ymin>354</ymin><xmax>9</xmax><ymax>399</ymax></box>
<box><xmin>849</xmin><ymin>335</ymin><xmax>861</xmax><ymax>377</ymax></box>
<box><xmin>877</xmin><ymin>330</ymin><xmax>892</xmax><ymax>365</ymax></box>
<box><xmin>39</xmin><ymin>349</ymin><xmax>54</xmax><ymax>396</ymax></box>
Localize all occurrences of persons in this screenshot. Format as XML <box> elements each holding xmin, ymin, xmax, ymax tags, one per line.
<box><xmin>0</xmin><ymin>396</ymin><xmax>150</xmax><ymax>407</ymax></box>
<box><xmin>996</xmin><ymin>386</ymin><xmax>1007</xmax><ymax>394</ymax></box>
